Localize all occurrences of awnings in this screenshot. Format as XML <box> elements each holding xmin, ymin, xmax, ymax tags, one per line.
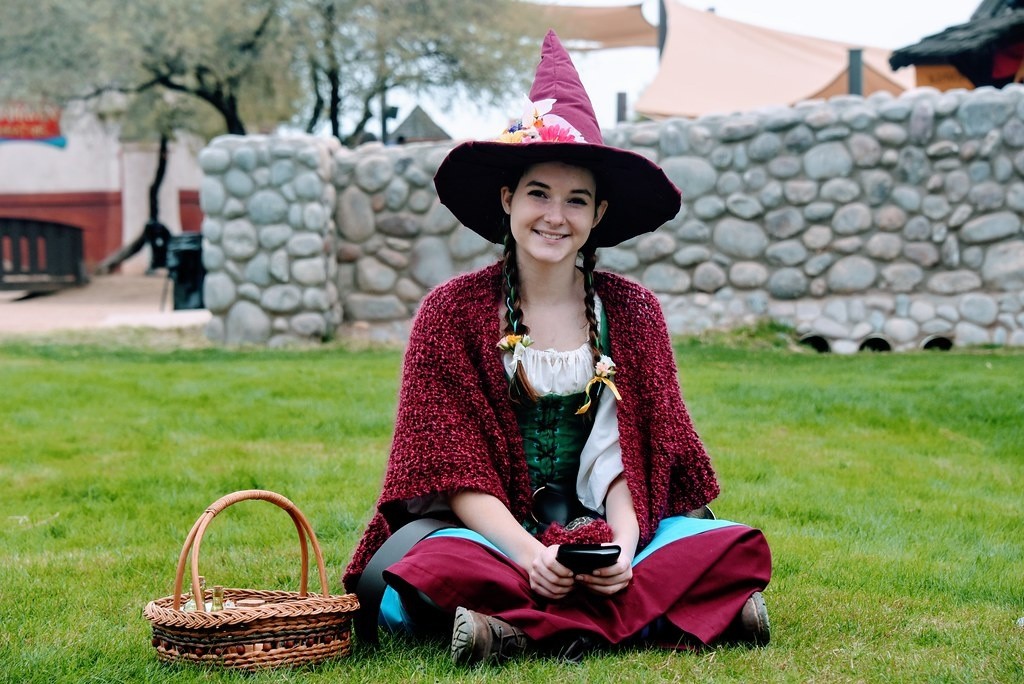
<box><xmin>888</xmin><ymin>12</ymin><xmax>1024</xmax><ymax>70</ymax></box>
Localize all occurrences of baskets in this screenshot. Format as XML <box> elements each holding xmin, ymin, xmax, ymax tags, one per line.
<box><xmin>143</xmin><ymin>490</ymin><xmax>360</xmax><ymax>673</ymax></box>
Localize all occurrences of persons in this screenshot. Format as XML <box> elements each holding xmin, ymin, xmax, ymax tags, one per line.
<box><xmin>345</xmin><ymin>32</ymin><xmax>773</xmax><ymax>667</ymax></box>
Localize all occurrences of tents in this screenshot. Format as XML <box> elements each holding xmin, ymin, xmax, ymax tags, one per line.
<box><xmin>377</xmin><ymin>0</ymin><xmax>917</xmax><ymax>148</ymax></box>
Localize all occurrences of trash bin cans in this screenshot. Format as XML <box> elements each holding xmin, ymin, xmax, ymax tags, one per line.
<box><xmin>164</xmin><ymin>233</ymin><xmax>204</xmax><ymax>311</ymax></box>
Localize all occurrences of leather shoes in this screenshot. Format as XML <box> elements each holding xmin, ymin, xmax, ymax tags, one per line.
<box><xmin>451</xmin><ymin>606</ymin><xmax>526</xmax><ymax>666</ymax></box>
<box><xmin>741</xmin><ymin>592</ymin><xmax>771</xmax><ymax>645</ymax></box>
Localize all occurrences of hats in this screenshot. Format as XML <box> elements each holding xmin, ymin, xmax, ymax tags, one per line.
<box><xmin>433</xmin><ymin>45</ymin><xmax>682</xmax><ymax>248</ymax></box>
